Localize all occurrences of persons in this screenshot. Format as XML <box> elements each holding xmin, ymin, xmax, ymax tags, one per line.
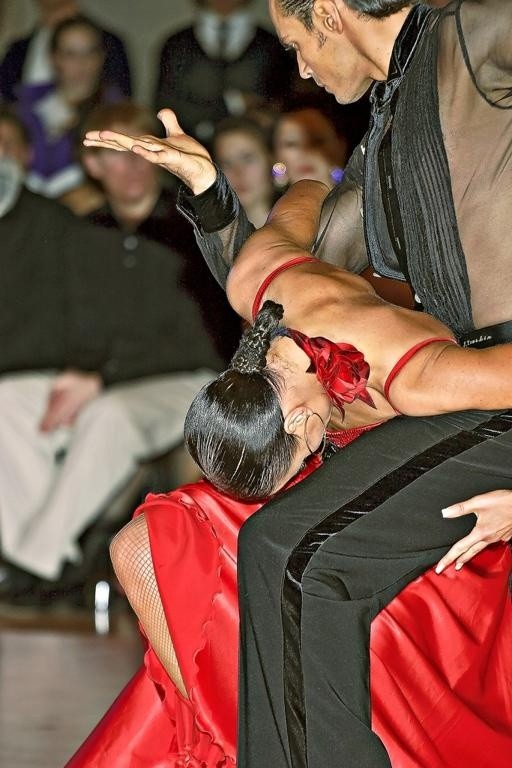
<box><xmin>257</xmin><ymin>94</ymin><xmax>355</xmax><ymax>208</ymax></box>
<box><xmin>1</xmin><ymin>101</ymin><xmax>254</xmax><ymax>618</ymax></box>
<box><xmin>3</xmin><ymin>117</ymin><xmax>96</xmax><ymax>371</ymax></box>
<box><xmin>73</xmin><ymin>0</ymin><xmax>512</xmax><ymax>768</ymax></box>
<box><xmin>53</xmin><ymin>160</ymin><xmax>512</xmax><ymax>768</ymax></box>
<box><xmin>0</xmin><ymin>1</ymin><xmax>134</xmax><ymax>116</ymax></box>
<box><xmin>149</xmin><ymin>114</ymin><xmax>295</xmax><ymax>365</ymax></box>
<box><xmin>151</xmin><ymin>0</ymin><xmax>326</xmax><ymax>142</ymax></box>
